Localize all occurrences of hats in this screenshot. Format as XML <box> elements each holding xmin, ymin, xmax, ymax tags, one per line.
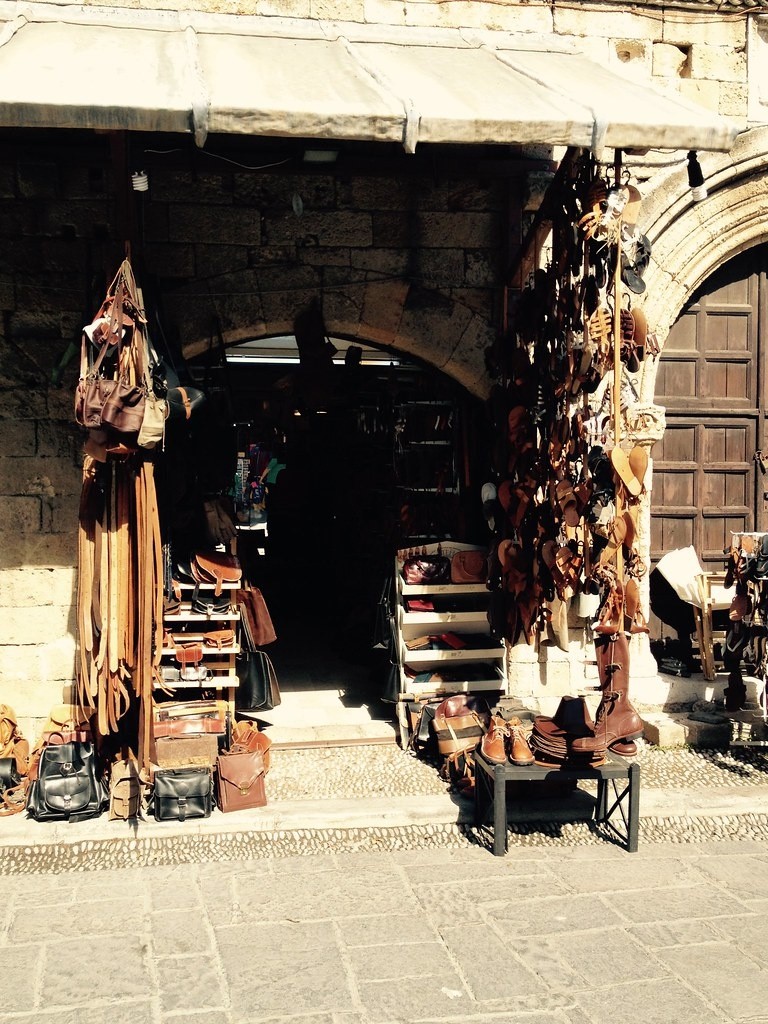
<box><xmin>528</xmin><ymin>696</ymin><xmax>608</xmax><ymax>769</ymax></box>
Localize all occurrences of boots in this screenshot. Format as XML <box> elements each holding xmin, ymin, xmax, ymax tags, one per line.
<box><xmin>570</xmin><ymin>633</ymin><xmax>644</xmax><ymax>757</ymax></box>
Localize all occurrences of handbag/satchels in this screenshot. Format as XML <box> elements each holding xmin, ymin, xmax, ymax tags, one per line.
<box><xmin>403</xmin><ymin>555</ymin><xmax>449</xmax><ymax>585</ymax></box>
<box><xmin>398</xmin><ymin>693</ymin><xmax>540</xmax><ymax>798</ymax></box>
<box><xmin>0</xmin><ymin>553</ymin><xmax>282</xmax><ymax>821</ymax></box>
<box><xmin>450</xmin><ymin>550</ymin><xmax>495</xmax><ymax>584</ymax></box>
<box><xmin>74</xmin><ymin>260</ymin><xmax>205</xmax><ymax>448</ymax></box>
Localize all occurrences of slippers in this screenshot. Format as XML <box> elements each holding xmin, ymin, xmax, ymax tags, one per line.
<box><xmin>472</xmin><ymin>188</ymin><xmax>651</xmax><ymax>654</ymax></box>
<box><xmin>716</xmin><ymin>532</ymin><xmax>768</xmax><ymax>713</ymax></box>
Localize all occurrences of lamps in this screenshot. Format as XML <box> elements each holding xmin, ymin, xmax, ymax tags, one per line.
<box><xmin>130</xmin><ymin>147</ymin><xmax>149</xmax><ymax>193</ymax></box>
<box><xmin>686</xmin><ymin>149</ymin><xmax>708</xmax><ymax>203</ymax></box>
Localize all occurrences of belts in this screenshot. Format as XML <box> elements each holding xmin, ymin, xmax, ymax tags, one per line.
<box><xmin>74</xmin><ymin>444</ymin><xmax>165</xmax><ymax>736</ymax></box>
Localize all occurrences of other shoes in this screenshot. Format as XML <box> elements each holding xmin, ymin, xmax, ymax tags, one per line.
<box><xmin>480</xmin><ymin>714</ymin><xmax>512</xmax><ymax>765</ymax></box>
<box><xmin>508</xmin><ymin>717</ymin><xmax>534</xmax><ymax>765</ymax></box>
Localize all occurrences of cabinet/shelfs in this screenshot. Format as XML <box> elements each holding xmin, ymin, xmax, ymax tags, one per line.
<box><xmin>522</xmin><ymin>152</ymin><xmax>642</xmax><ymax>627</ymax></box>
<box><xmin>150</xmin><ymin>522</ymin><xmax>242</xmax><ymax>760</ymax></box>
<box><xmin>343</xmin><ymin>400</ymin><xmax>507</xmax><ymax>750</ymax></box>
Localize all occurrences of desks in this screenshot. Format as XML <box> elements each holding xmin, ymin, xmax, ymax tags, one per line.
<box><xmin>471</xmin><ymin>749</ymin><xmax>640</xmax><ymax>857</ymax></box>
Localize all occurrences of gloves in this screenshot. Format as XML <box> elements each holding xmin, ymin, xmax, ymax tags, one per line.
<box><xmin>203</xmin><ymin>500</ymin><xmax>240</xmax><ymax>544</ymax></box>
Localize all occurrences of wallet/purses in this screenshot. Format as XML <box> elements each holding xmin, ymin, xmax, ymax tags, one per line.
<box><xmin>403</xmin><ymin>599</ymin><xmax>498</xmax><ymax>683</ymax></box>
